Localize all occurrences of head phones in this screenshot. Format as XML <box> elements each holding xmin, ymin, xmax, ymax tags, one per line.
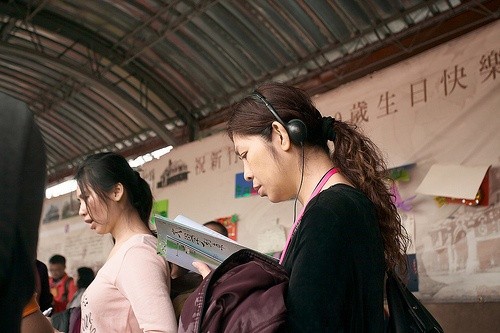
<box><xmin>251</xmin><ymin>90</ymin><xmax>307</xmax><ymax>146</ymax></box>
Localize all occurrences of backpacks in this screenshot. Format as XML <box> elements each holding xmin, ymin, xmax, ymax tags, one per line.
<box><xmin>385</xmin><ymin>257</ymin><xmax>444</xmax><ymax>333</ymax></box>
